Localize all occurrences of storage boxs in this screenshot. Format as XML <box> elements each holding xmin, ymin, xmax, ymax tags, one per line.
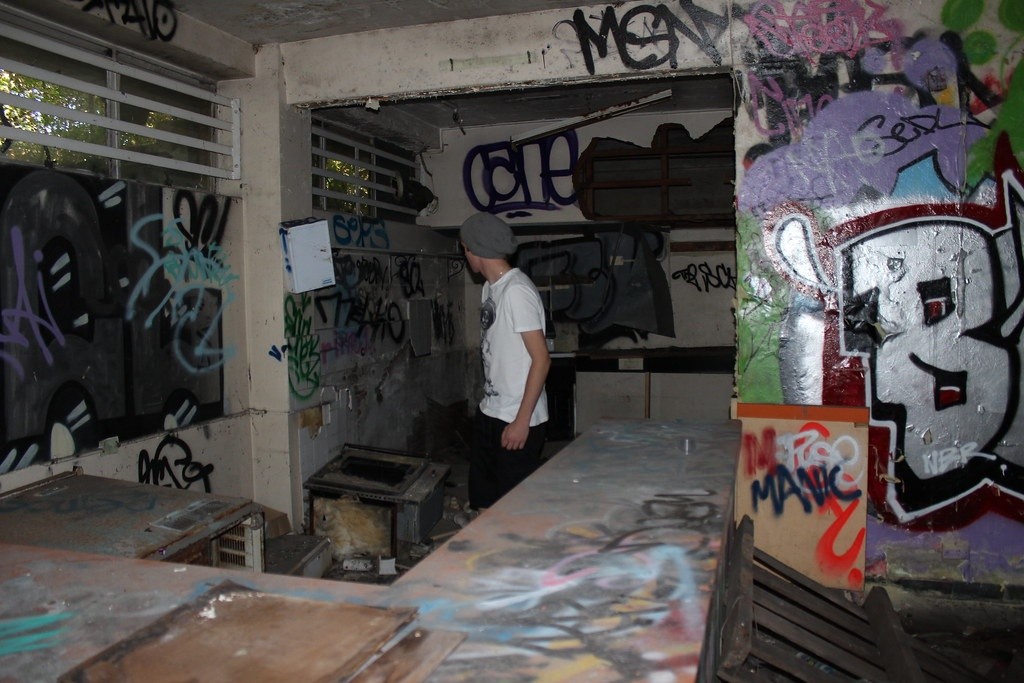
<box><xmin>397</xmin><ymin>462</ymin><xmax>452</xmax><ymax>543</ymax></box>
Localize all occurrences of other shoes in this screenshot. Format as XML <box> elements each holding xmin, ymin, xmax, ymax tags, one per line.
<box><xmin>454</xmin><ymin>509</ymin><xmax>484</xmax><ymax>528</ymax></box>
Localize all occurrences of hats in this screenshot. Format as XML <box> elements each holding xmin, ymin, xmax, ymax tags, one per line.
<box><xmin>460</xmin><ymin>212</ymin><xmax>517</xmax><ymax>258</ymax></box>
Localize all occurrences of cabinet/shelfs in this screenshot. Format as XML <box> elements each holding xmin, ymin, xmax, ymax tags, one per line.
<box><xmin>573</xmin><ymin>346</ymin><xmax>736</xmax><ymax>438</ymax></box>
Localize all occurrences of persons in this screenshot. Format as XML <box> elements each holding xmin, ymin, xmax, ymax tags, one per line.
<box><xmin>460</xmin><ymin>211</ymin><xmax>552</xmax><ymax>503</ymax></box>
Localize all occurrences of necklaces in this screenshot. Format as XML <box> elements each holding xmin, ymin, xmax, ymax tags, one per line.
<box><xmin>496</xmin><ymin>267</ymin><xmax>505</xmax><ymax>281</ymax></box>
